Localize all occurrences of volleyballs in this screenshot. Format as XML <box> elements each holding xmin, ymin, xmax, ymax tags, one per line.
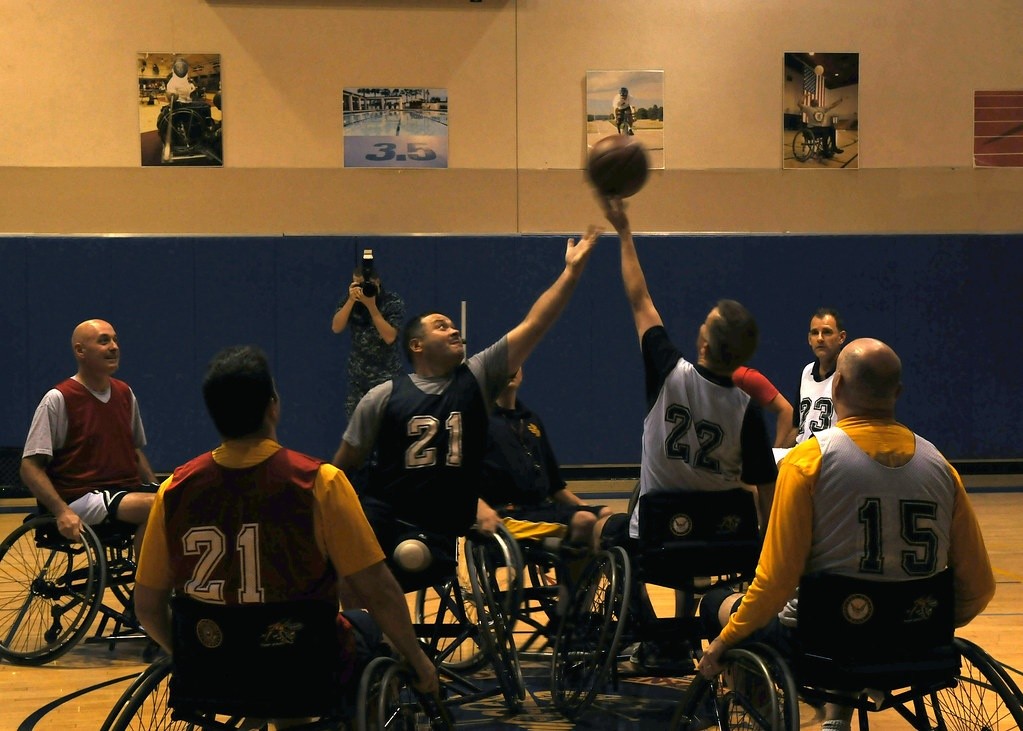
<box><xmin>814</xmin><ymin>64</ymin><xmax>825</xmax><ymax>75</ymax></box>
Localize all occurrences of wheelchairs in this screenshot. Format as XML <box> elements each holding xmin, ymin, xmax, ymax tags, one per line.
<box><xmin>0</xmin><ymin>481</ymin><xmax>164</xmax><ymax>670</ymax></box>
<box><xmin>156</xmin><ymin>94</ymin><xmax>218</xmax><ymax>162</ymax></box>
<box><xmin>792</xmin><ymin>121</ymin><xmax>830</xmax><ymax>161</ymax></box>
<box><xmin>94</xmin><ymin>514</ymin><xmax>1023</xmax><ymax>730</ymax></box>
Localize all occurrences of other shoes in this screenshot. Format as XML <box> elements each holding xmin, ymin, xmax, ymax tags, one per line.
<box><xmin>831</xmin><ymin>146</ymin><xmax>843</xmax><ymax>153</ymax></box>
<box><xmin>630</xmin><ymin>639</ymin><xmax>679</xmax><ymax>674</ymax></box>
<box><xmin>823</xmin><ymin>149</ymin><xmax>832</xmax><ymax>156</ymax></box>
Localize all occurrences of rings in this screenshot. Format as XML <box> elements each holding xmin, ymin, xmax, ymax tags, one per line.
<box><xmin>706</xmin><ymin>664</ymin><xmax>711</xmax><ymax>669</ymax></box>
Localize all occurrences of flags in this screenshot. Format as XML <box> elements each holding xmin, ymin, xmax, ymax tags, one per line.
<box><xmin>802</xmin><ymin>65</ymin><xmax>827</xmax><ymax>124</ymax></box>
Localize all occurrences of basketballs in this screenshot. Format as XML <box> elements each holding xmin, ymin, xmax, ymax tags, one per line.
<box><xmin>587</xmin><ymin>133</ymin><xmax>652</xmax><ymax>200</ymax></box>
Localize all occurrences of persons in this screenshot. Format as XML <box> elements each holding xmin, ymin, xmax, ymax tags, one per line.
<box><xmin>21</xmin><ymin>319</ymin><xmax>166</xmax><ymax>662</ymax></box>
<box><xmin>332</xmin><ymin>253</ymin><xmax>406</xmax><ymax>423</ymax></box>
<box><xmin>165</xmin><ymin>59</ymin><xmax>218</xmax><ymax>140</ymax></box>
<box><xmin>613</xmin><ymin>87</ymin><xmax>636</xmax><ymax>135</ymax></box>
<box><xmin>134</xmin><ymin>347</ymin><xmax>438</xmax><ymax>731</ymax></box>
<box><xmin>334</xmin><ymin>227</ymin><xmax>607</xmax><ymax>611</ymax></box>
<box><xmin>776</xmin><ymin>310</ymin><xmax>846</xmax><ymax>471</ymax></box>
<box><xmin>797</xmin><ymin>94</ymin><xmax>846</xmax><ymax>156</ymax></box>
<box><xmin>590</xmin><ymin>190</ymin><xmax>793</xmax><ymax>678</ymax></box>
<box><xmin>477</xmin><ymin>367</ymin><xmax>657</xmax><ymax>647</ymax></box>
<box><xmin>697</xmin><ymin>338</ymin><xmax>996</xmax><ymax>731</ymax></box>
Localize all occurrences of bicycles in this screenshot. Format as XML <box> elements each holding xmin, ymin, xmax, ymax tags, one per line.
<box><xmin>615</xmin><ymin>114</ymin><xmax>636</xmax><ymax>138</ymax></box>
